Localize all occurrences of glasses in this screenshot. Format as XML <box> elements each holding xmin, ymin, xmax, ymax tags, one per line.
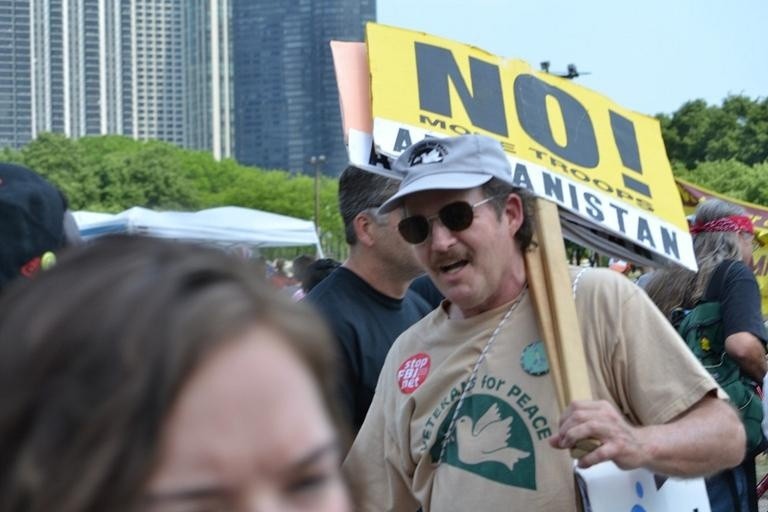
<box><xmin>397</xmin><ymin>196</ymin><xmax>494</xmax><ymax>244</ymax></box>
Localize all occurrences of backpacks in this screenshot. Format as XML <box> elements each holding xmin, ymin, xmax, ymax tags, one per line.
<box><xmin>675</xmin><ymin>257</ymin><xmax>768</xmax><ymax>458</ymax></box>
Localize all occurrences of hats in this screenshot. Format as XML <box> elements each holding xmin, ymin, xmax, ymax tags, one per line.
<box><xmin>377</xmin><ymin>134</ymin><xmax>517</xmax><ymax>215</ymax></box>
<box><xmin>1</xmin><ymin>157</ymin><xmax>68</xmax><ymax>270</ymax></box>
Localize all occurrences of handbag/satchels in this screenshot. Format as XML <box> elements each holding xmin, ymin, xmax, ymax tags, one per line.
<box><xmin>573</xmin><ymin>450</ymin><xmax>713</xmax><ymax>511</ymax></box>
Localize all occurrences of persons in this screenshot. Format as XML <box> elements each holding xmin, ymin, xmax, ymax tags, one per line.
<box><xmin>297</xmin><ymin>166</ymin><xmax>438</xmax><ymax>434</ymax></box>
<box><xmin>227</xmin><ymin>244</ymin><xmax>341</xmax><ymax>301</ymax></box>
<box><xmin>579</xmin><ymin>255</ymin><xmax>697</xmax><ymax>326</ymax></box>
<box><xmin>677</xmin><ymin>197</ymin><xmax>767</xmax><ymax>512</ymax></box>
<box><xmin>412</xmin><ymin>272</ymin><xmax>446</xmax><ymax>309</ymax></box>
<box><xmin>335</xmin><ymin>130</ymin><xmax>746</xmax><ymax>512</ymax></box>
<box><xmin>0</xmin><ymin>234</ymin><xmax>359</xmax><ymax>511</ymax></box>
<box><xmin>2</xmin><ymin>163</ymin><xmax>83</xmax><ymax>302</ymax></box>
